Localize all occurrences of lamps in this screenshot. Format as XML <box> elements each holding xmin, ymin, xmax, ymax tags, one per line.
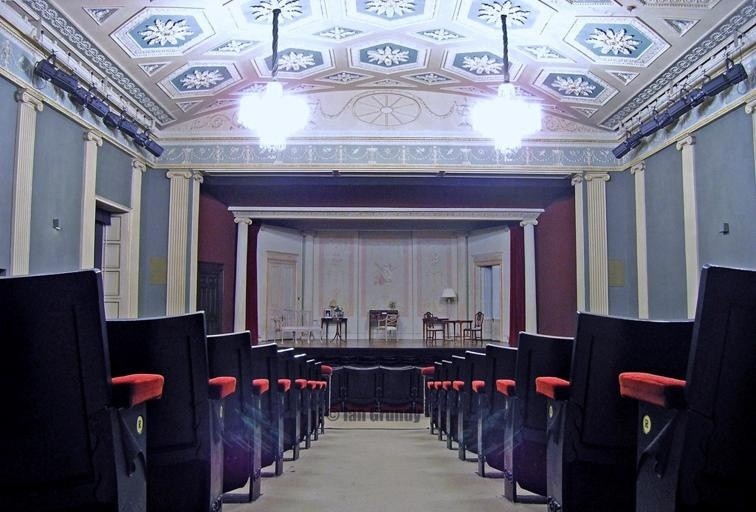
<box><xmin>464</xmin><ymin>13</ymin><xmax>544</xmax><ymax>158</ymax></box>
<box><xmin>32</xmin><ymin>51</ymin><xmax>165</xmax><ymax>158</ymax></box>
<box><xmin>232</xmin><ymin>8</ymin><xmax>313</xmax><ymax>155</ymax></box>
<box><xmin>610</xmin><ymin>56</ymin><xmax>749</xmax><ymax>160</ymax></box>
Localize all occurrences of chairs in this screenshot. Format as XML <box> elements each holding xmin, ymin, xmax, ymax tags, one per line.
<box><xmin>0</xmin><ymin>267</ymin><xmax>333</xmax><ymax>511</ymax></box>
<box><xmin>329</xmin><ymin>362</ymin><xmax>427</xmax><ymax>414</ymax></box>
<box><xmin>422</xmin><ymin>260</ymin><xmax>756</xmax><ymax>509</ymax></box>
<box><xmin>376</xmin><ymin>313</ymin><xmax>401</xmax><ymax>345</ymax></box>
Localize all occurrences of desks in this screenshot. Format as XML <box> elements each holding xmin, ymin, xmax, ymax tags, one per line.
<box><xmin>319</xmin><ymin>317</ymin><xmax>348</xmax><ymax>343</ymax></box>
<box><xmin>368</xmin><ymin>309</ymin><xmax>399</xmax><ymax>342</ymax></box>
